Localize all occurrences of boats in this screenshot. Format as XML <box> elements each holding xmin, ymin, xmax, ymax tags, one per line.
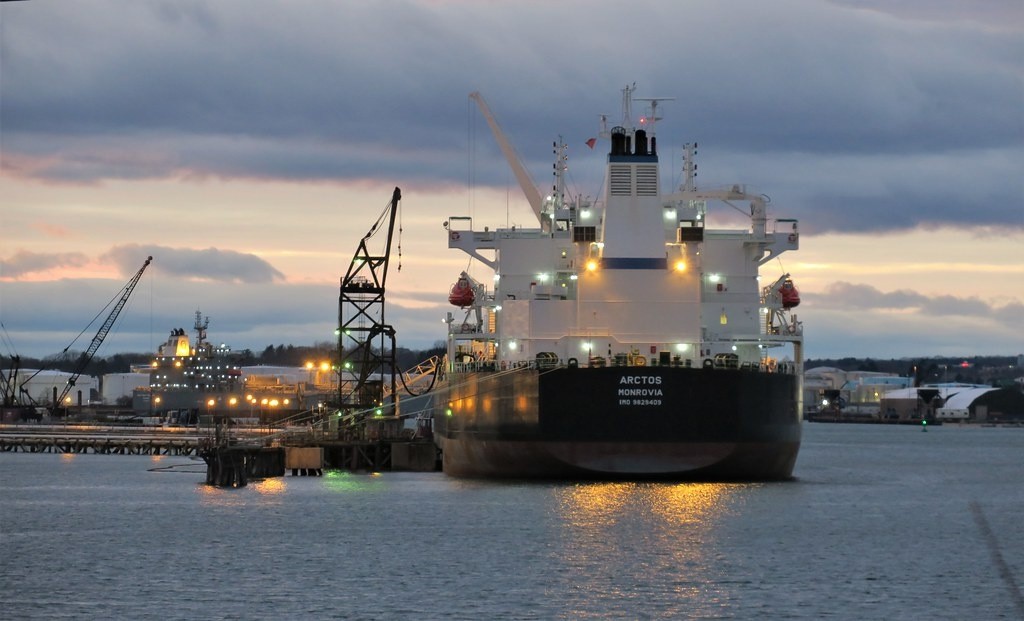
<box><xmin>435</xmin><ymin>82</ymin><xmax>811</xmax><ymax>485</ymax></box>
<box><xmin>779</xmin><ymin>279</ymin><xmax>802</xmax><ymax>307</ymax></box>
<box><xmin>449</xmin><ymin>277</ymin><xmax>475</xmax><ymax>306</ymax></box>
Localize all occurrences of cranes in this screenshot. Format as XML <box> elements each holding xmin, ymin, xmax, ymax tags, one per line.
<box><xmin>331</xmin><ymin>185</ymin><xmax>403</xmax><ymax>418</ymax></box>
<box><xmin>17</xmin><ymin>257</ymin><xmax>155</xmax><ymax>423</ymax></box>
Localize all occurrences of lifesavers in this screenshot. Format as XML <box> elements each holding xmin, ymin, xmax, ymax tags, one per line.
<box><xmin>788</xmin><ymin>325</ymin><xmax>796</xmax><ymax>333</ymax></box>
<box><xmin>462</xmin><ymin>324</ymin><xmax>470</xmax><ymax>331</ymax></box>
<box><xmin>788</xmin><ymin>234</ymin><xmax>797</xmax><ymax>242</ymax></box>
<box><xmin>634</xmin><ymin>355</ymin><xmax>646</xmax><ymax>366</ymax></box>
<box><xmin>451</xmin><ymin>232</ymin><xmax>460</xmax><ymax>239</ymax></box>
